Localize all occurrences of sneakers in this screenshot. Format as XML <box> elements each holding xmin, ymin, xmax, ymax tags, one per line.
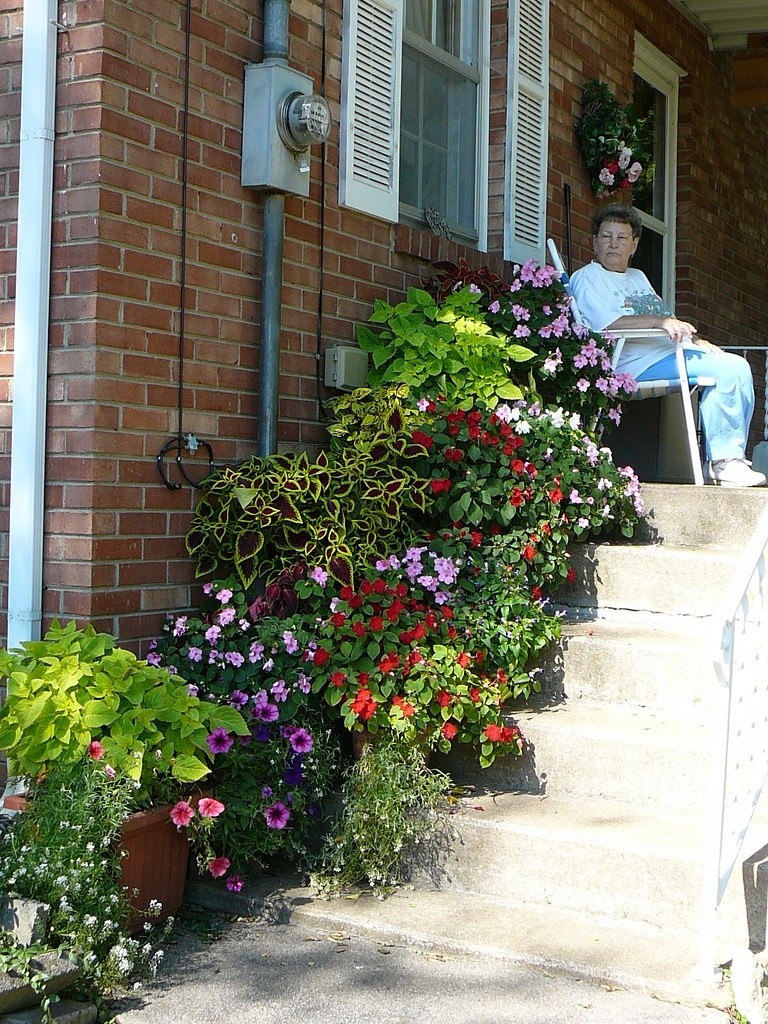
<box><xmin>702</xmin><ymin>458</ymin><xmax>767</xmax><ymax>486</ymax></box>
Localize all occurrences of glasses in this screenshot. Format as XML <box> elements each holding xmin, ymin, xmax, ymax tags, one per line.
<box><xmin>597</xmin><ymin>231</ymin><xmax>633</xmax><ymax>244</ymax></box>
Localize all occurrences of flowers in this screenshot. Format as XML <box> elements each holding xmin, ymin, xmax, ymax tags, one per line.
<box><xmin>1</xmin><ymin>258</ymin><xmax>656</xmax><ymax>993</ymax></box>
<box><xmin>577</xmin><ymin>81</ymin><xmax>652</xmax><ymax>199</ymax></box>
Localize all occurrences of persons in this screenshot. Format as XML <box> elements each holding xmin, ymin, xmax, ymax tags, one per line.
<box><xmin>568</xmin><ymin>201</ymin><xmax>768</xmax><ymax>487</ymax></box>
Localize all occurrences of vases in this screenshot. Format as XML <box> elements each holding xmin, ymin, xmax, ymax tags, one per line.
<box><xmin>352</xmin><ymin>728</ymin><xmax>430</xmax><ymax>770</ymax></box>
<box><xmin>4</xmin><ymin>791</ymin><xmax>213</xmax><ymax>936</ymax></box>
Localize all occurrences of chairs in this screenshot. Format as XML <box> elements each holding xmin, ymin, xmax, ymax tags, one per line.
<box><xmin>547</xmin><ymin>239</ymin><xmax>704</xmax><ymax>486</ymax></box>
